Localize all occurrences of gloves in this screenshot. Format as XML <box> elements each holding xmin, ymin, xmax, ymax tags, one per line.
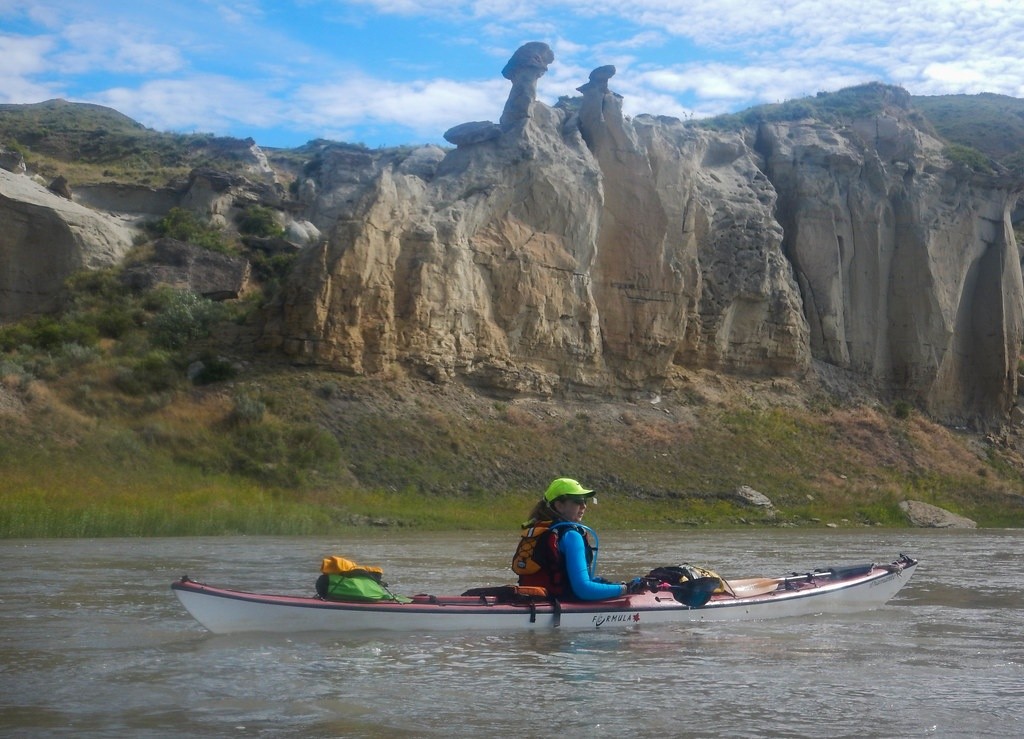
<box><xmin>625</xmin><ymin>580</ymin><xmax>646</xmax><ymax>594</ymax></box>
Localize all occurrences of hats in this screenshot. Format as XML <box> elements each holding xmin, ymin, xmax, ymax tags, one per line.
<box><xmin>544</xmin><ymin>478</ymin><xmax>596</xmax><ymax>503</ymax></box>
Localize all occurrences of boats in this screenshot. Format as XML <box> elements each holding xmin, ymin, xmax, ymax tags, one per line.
<box><xmin>171</xmin><ymin>552</ymin><xmax>921</xmax><ymax>634</ymax></box>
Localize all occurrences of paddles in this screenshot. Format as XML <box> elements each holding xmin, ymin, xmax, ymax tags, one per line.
<box><xmin>721</xmin><ymin>562</ymin><xmax>870</xmax><ymax>599</ymax></box>
<box><xmin>646</xmin><ymin>576</ymin><xmax>721</xmax><ymax>606</ymax></box>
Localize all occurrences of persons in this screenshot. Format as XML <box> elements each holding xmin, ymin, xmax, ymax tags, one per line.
<box><xmin>512</xmin><ymin>478</ymin><xmax>649</xmax><ymax>602</ymax></box>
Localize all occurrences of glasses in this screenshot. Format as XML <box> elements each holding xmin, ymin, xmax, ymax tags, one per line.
<box><xmin>576</xmin><ymin>496</ymin><xmax>587</xmax><ymax>503</ymax></box>
